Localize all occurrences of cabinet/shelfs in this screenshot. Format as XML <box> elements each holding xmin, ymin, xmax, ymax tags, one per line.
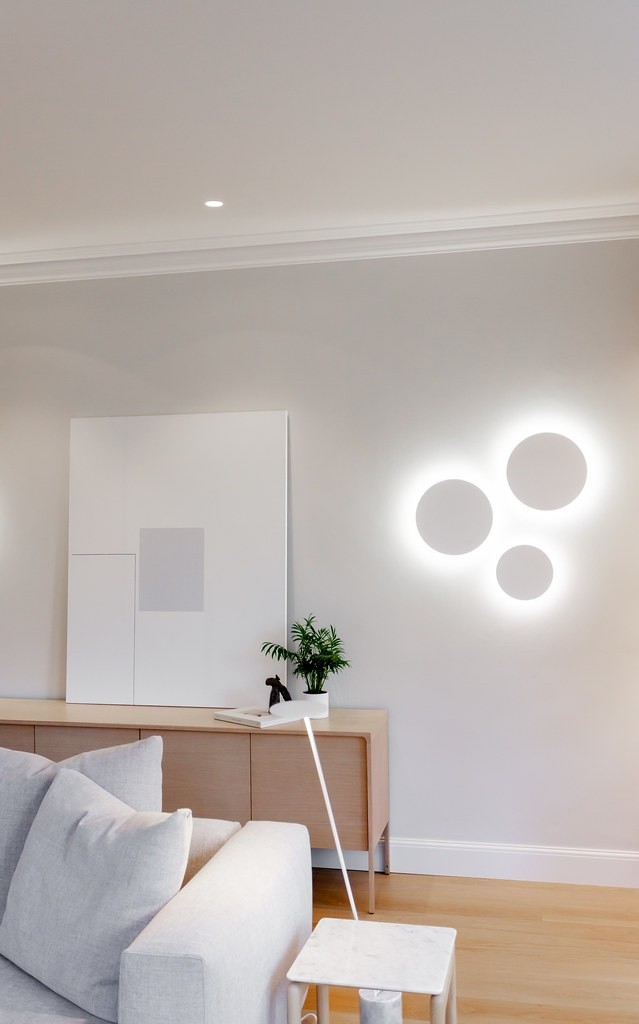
<box><xmin>0</xmin><ymin>698</ymin><xmax>391</xmax><ymax>915</ymax></box>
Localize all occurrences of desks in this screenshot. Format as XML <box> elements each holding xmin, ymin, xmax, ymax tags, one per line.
<box><xmin>287</xmin><ymin>917</ymin><xmax>457</xmax><ymax>1024</ymax></box>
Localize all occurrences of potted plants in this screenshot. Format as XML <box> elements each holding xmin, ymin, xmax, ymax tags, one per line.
<box><xmin>259</xmin><ymin>612</ymin><xmax>348</xmax><ymax>717</ymax></box>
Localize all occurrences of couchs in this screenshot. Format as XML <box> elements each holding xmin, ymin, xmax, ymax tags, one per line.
<box><xmin>0</xmin><ymin>812</ymin><xmax>314</xmax><ymax>1024</ymax></box>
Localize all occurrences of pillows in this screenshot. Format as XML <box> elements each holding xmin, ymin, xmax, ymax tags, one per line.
<box><xmin>0</xmin><ymin>731</ymin><xmax>163</xmax><ymax>924</ymax></box>
<box><xmin>0</xmin><ymin>770</ymin><xmax>193</xmax><ymax>1020</ymax></box>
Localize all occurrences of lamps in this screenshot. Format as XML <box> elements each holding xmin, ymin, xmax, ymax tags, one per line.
<box><xmin>269</xmin><ymin>700</ymin><xmax>404</xmax><ymax>1024</ymax></box>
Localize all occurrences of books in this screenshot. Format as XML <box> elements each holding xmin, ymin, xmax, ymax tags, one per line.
<box><xmin>213</xmin><ymin>707</ymin><xmax>302</xmax><ymax>729</ymax></box>
